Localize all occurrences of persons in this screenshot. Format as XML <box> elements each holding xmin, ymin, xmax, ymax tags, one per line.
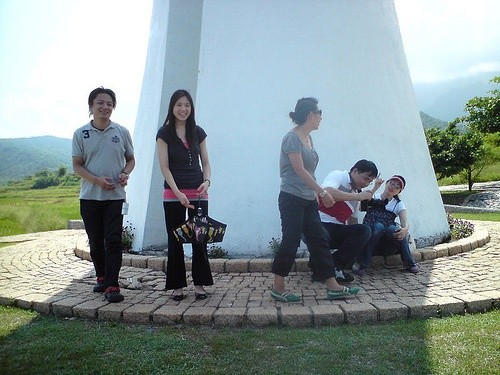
<box><xmin>316</xmin><ymin>159</ymin><xmax>419</xmax><ymax>282</ymax></box>
<box><xmin>270</xmin><ymin>98</ymin><xmax>360</xmax><ymax>302</ymax></box>
<box><xmin>72</xmin><ymin>86</ymin><xmax>135</xmax><ymax>303</ymax></box>
<box><xmin>156</xmin><ymin>89</ymin><xmax>227</xmax><ymax>301</ymax></box>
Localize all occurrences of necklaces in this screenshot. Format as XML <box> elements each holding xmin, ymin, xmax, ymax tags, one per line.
<box><xmin>298</xmin><ymin>125</ymin><xmax>309</xmax><ymax>143</ymax></box>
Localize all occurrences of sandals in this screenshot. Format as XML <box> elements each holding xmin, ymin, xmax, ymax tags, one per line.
<box><xmin>92</xmin><ymin>277</ymin><xmax>106</xmax><ymax>291</ymax></box>
<box><xmin>105</xmin><ymin>286</ymin><xmax>124</xmax><ymax>302</ymax></box>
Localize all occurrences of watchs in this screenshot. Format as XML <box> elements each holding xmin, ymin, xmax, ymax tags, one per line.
<box><xmin>122</xmin><ymin>171</ymin><xmax>130</xmax><ymax>180</ymax></box>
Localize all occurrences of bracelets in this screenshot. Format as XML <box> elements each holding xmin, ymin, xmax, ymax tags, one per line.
<box><xmin>205</xmin><ymin>179</ymin><xmax>210</xmax><ymax>186</ymax></box>
<box><xmin>318</xmin><ymin>190</ymin><xmax>327</xmax><ymax>198</ymax></box>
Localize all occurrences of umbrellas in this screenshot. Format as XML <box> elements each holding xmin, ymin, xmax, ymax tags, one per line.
<box><xmin>173</xmin><ymin>192</ymin><xmax>227</xmax><ymax>243</ymax></box>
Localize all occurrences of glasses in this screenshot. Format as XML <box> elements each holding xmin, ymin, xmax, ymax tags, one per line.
<box><xmin>389</xmin><ymin>179</ymin><xmax>401</xmax><ymax>191</ymax></box>
<box><xmin>357</xmin><ymin>171</ymin><xmax>375</xmax><ymax>186</ymax></box>
<box><xmin>307</xmin><ymin>110</ymin><xmax>322</xmax><ymax>116</ymax></box>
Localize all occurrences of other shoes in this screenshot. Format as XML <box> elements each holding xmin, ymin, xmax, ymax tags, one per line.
<box><xmin>352</xmin><ymin>262</ymin><xmax>365</xmax><ymax>276</ymax></box>
<box><xmin>195</xmin><ymin>292</ymin><xmax>208</xmax><ymax>299</ymax></box>
<box><xmin>271</xmin><ymin>288</ymin><xmax>301</xmax><ymax>302</ymax></box>
<box><xmin>172</xmin><ymin>293</ymin><xmax>183</xmax><ymax>300</ymax></box>
<box><xmin>327</xmin><ymin>285</ymin><xmax>359</xmax><ymax>300</ymax></box>
<box><xmin>334</xmin><ymin>265</ymin><xmax>345</xmax><ymax>281</ymax></box>
<box><xmin>410</xmin><ymin>263</ymin><xmax>420</xmax><ymax>272</ymax></box>
